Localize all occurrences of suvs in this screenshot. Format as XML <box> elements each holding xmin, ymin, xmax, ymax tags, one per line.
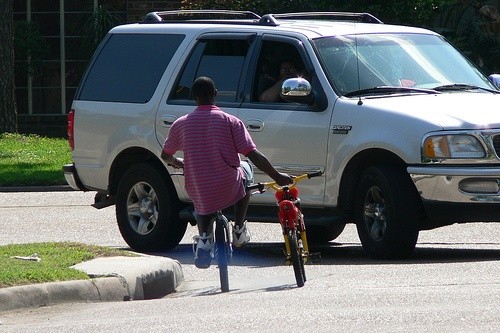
<box><xmin>63</xmin><ymin>8</ymin><xmax>500</xmax><ymax>261</ymax></box>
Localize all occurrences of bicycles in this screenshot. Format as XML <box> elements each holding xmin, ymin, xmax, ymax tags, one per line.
<box><xmin>246</xmin><ymin>170</ymin><xmax>322</xmax><ymax>287</ymax></box>
<box><xmin>166</xmin><ymin>160</ymin><xmax>246</xmax><ymax>293</ymax></box>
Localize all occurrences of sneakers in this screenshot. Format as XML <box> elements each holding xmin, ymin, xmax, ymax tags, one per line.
<box><xmin>191</xmin><ymin>230</ymin><xmax>214</xmax><ymax>269</ymax></box>
<box><xmin>230</xmin><ymin>220</ymin><xmax>250</xmax><ymax>249</ymax></box>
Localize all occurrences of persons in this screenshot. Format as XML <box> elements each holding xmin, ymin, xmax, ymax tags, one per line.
<box><xmin>161</xmin><ymin>77</ymin><xmax>295</xmax><ymax>269</ymax></box>
<box><xmin>258</xmin><ymin>58</ymin><xmax>307</xmax><ymax>103</ymax></box>
